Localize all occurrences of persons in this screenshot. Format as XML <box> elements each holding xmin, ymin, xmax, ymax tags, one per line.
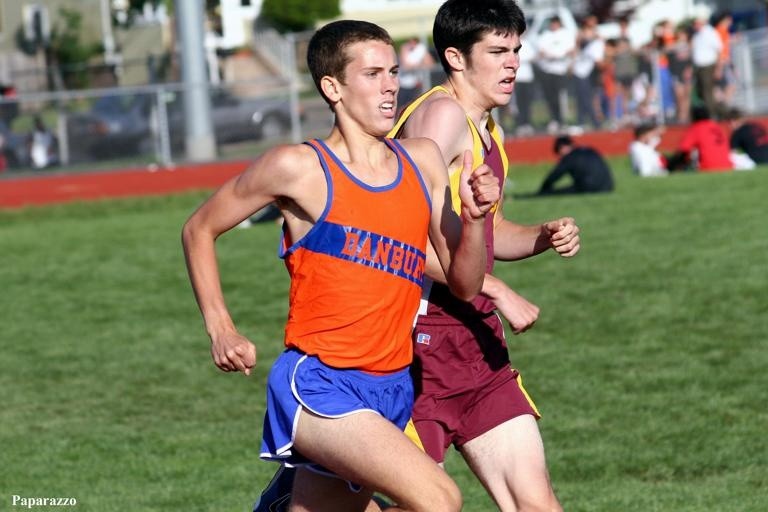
<box><xmin>397</xmin><ymin>37</ymin><xmax>436</xmax><ymax>116</ymax></box>
<box><xmin>181</xmin><ymin>19</ymin><xmax>502</xmax><ymax>512</ymax></box>
<box><xmin>255</xmin><ymin>1</ymin><xmax>580</xmax><ymax>512</ymax></box>
<box><xmin>498</xmin><ymin>0</ymin><xmax>768</xmax><ymax>195</ymax></box>
